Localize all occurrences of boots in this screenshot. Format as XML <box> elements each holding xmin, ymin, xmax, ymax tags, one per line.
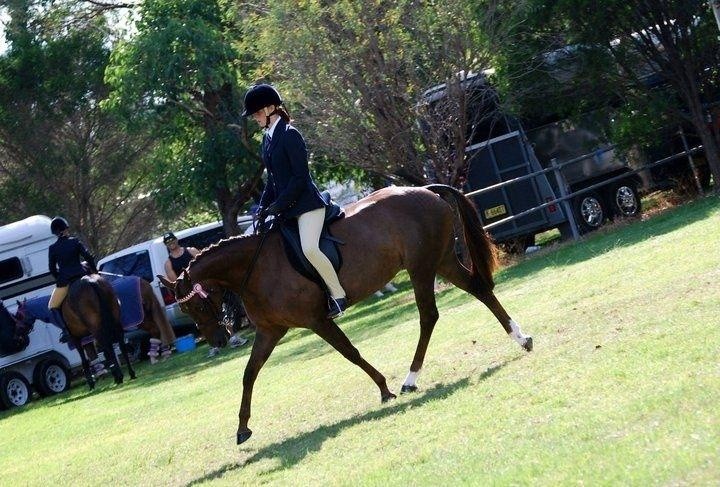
<box><xmin>51</xmin><ymin>307</ymin><xmax>72</xmax><ymax>343</ymax></box>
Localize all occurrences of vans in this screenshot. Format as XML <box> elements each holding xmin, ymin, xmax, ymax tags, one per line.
<box><xmin>0</xmin><ymin>215</ymin><xmax>94</xmax><ymax>407</ymax></box>
<box><xmin>96</xmin><ymin>215</ymin><xmax>259</xmax><ymax>340</ymax></box>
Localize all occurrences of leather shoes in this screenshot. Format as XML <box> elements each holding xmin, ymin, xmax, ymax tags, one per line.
<box><xmin>326</xmin><ymin>298</ymin><xmax>347</xmax><ymax>320</ymax></box>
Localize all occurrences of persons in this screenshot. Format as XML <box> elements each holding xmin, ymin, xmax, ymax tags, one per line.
<box><xmin>48</xmin><ymin>217</ymin><xmax>98</xmax><ymax>343</ymax></box>
<box><xmin>241</xmin><ymin>84</ymin><xmax>351</xmax><ymax>319</ymax></box>
<box><xmin>164</xmin><ymin>233</ymin><xmax>249</xmax><ymax>358</ymax></box>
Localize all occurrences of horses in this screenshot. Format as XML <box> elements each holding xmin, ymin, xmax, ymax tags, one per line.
<box><xmin>14</xmin><ymin>273</ymin><xmax>178</xmax><ymax>379</ymax></box>
<box><xmin>156</xmin><ymin>184</ymin><xmax>533</xmax><ymax>444</ymax></box>
<box><xmin>60</xmin><ymin>274</ymin><xmax>139</xmax><ymax>391</ymax></box>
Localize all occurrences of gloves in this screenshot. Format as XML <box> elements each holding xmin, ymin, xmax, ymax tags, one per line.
<box><xmin>253</xmin><ymin>205</ymin><xmax>282</xmax><ymax>235</ymax></box>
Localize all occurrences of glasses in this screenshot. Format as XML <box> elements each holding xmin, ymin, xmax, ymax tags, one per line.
<box><xmin>166</xmin><ymin>238</ymin><xmax>174</xmax><ymax>246</ymax></box>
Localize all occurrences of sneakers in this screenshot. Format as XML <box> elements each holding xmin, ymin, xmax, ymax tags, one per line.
<box><xmin>229</xmin><ymin>336</ymin><xmax>249</xmax><ymax>349</ymax></box>
<box><xmin>206</xmin><ymin>346</ymin><xmax>221</xmax><ymax>359</ymax></box>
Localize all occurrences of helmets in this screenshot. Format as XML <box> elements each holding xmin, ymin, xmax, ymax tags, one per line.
<box><xmin>241</xmin><ymin>84</ymin><xmax>281</xmax><ymax>117</ymax></box>
<box><xmin>51</xmin><ymin>217</ymin><xmax>69</xmax><ymax>234</ymax></box>
<box><xmin>163</xmin><ymin>233</ymin><xmax>177</xmax><ymax>243</ymax></box>
